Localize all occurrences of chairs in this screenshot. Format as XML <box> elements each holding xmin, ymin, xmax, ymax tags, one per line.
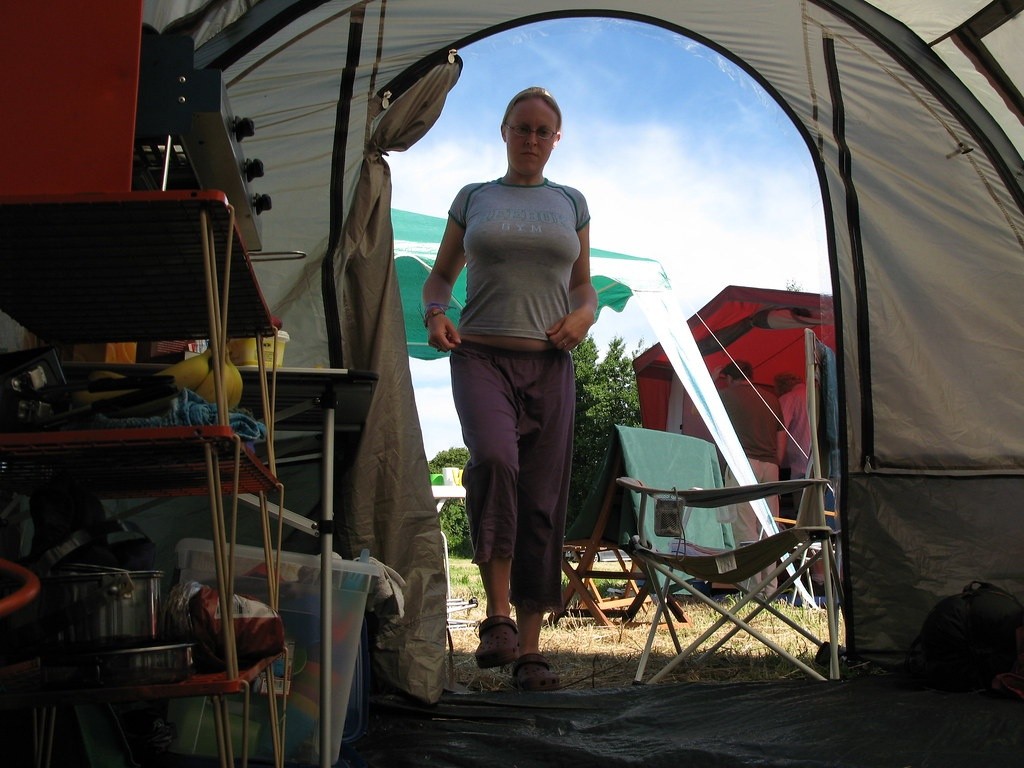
<box><xmin>740</xmin><ymin>510</ymin><xmax>838</xmax><ymax>607</ymax></box>
<box><xmin>614</xmin><ymin>329</ymin><xmax>846</xmax><ymax>686</ymax></box>
<box><xmin>545</xmin><ymin>424</ymin><xmax>717</xmax><ymax>631</ymax></box>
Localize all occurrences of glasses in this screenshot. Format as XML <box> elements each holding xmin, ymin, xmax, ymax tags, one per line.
<box><xmin>505</xmin><ymin>122</ymin><xmax>557</xmax><ymax>141</ymax></box>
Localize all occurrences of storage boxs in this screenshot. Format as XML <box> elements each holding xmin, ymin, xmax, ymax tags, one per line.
<box><xmin>163</xmin><ymin>538</ymin><xmax>383</xmax><ymax>768</ymax></box>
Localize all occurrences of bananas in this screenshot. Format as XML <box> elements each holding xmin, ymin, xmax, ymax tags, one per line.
<box><xmin>78</xmin><ymin>343</ymin><xmax>243</xmax><ymax>411</ymax></box>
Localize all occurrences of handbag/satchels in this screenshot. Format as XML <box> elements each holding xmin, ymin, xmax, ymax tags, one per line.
<box><xmin>901</xmin><ymin>580</ymin><xmax>1024</xmax><ymax>692</ymax></box>
<box><xmin>187</xmin><ymin>584</ymin><xmax>287</xmax><ymax>671</ymax></box>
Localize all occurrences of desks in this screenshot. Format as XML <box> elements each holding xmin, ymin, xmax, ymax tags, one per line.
<box><xmin>432</xmin><ymin>485</ymin><xmax>477</xmax><ymax>629</ymax></box>
<box><xmin>61</xmin><ymin>361</ymin><xmax>380</xmax><ymax>767</ymax></box>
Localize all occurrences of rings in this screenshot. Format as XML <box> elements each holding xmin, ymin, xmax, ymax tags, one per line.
<box><xmin>562</xmin><ymin>340</ymin><xmax>568</xmax><ymax>348</ymax></box>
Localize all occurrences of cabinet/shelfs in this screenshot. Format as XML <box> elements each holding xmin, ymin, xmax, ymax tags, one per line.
<box><xmin>0</xmin><ymin>0</ymin><xmax>290</xmax><ymax>768</ymax></box>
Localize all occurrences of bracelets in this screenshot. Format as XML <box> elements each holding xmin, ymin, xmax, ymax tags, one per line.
<box><xmin>422</xmin><ymin>306</ymin><xmax>447</xmax><ymax>328</ymax></box>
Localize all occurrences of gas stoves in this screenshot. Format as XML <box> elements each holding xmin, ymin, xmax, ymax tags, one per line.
<box><xmin>138</xmin><ymin>67</ymin><xmax>273</xmax><ymax>250</ymax></box>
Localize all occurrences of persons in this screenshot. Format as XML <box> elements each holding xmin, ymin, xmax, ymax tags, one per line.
<box><xmin>775</xmin><ymin>372</ymin><xmax>826</xmax><ymax>595</ymax></box>
<box><xmin>713</xmin><ymin>360</ymin><xmax>787</xmax><ymax>602</ymax></box>
<box><xmin>423</xmin><ymin>86</ymin><xmax>597</xmax><ymax>691</ymax></box>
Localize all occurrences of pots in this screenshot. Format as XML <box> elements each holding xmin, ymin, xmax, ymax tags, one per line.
<box><xmin>42</xmin><ymin>569</ymin><xmax>166</xmax><ymax>642</ymax></box>
<box><xmin>87</xmin><ymin>643</ymin><xmax>195</xmax><ymax>686</ymax></box>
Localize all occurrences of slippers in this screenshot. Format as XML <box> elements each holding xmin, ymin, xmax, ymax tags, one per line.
<box><xmin>512</xmin><ymin>653</ymin><xmax>561</xmax><ymax>692</ymax></box>
<box><xmin>475</xmin><ymin>614</ymin><xmax>518</xmax><ymax>669</ymax></box>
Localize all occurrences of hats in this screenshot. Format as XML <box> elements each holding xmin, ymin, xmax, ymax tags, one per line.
<box><xmin>720</xmin><ymin>359</ymin><xmax>753</xmax><ymax>380</ymax></box>
